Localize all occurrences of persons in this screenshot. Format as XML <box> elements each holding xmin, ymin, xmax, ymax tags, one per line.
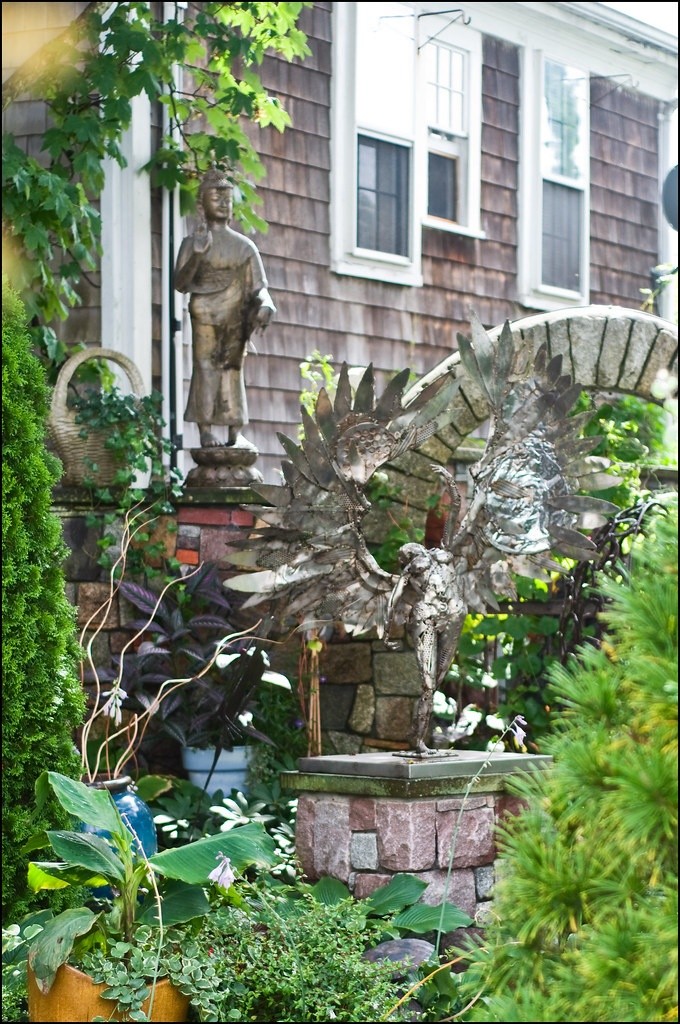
<box><xmin>175</xmin><ymin>170</ymin><xmax>279</xmax><ymax>448</ymax></box>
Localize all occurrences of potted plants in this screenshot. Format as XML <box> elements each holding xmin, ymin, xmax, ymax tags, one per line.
<box><xmin>80</xmin><ymin>560</ymin><xmax>276</xmax><ymax>803</ymax></box>
<box><xmin>23</xmin><ymin>771</ymin><xmax>282</xmax><ymax>1022</ymax></box>
